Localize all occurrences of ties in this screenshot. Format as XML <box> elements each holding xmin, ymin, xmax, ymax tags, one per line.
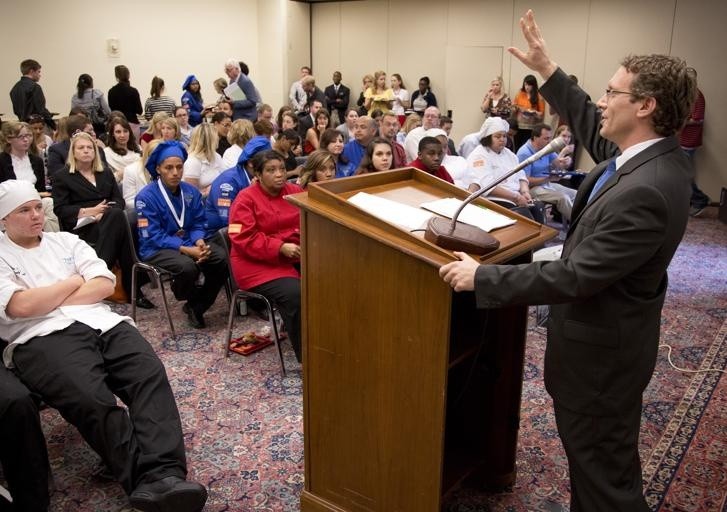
<box><xmin>586</xmin><ymin>160</ymin><xmax>618</xmax><ymax>205</ymax></box>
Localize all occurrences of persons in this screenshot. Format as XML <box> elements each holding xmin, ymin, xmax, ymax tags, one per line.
<box><xmin>479</xmin><ymin>75</ymin><xmax>512</xmax><ymax>118</ymax></box>
<box><xmin>356</xmin><ymin>69</ymin><xmax>438</xmax><ymax>126</ymax></box>
<box><xmin>10</xmin><ymin>60</ymin><xmax>56</xmax><ymax>128</ymax></box>
<box><xmin>299</xmin><ymin>75</ymin><xmax>328</xmax><ymax>109</ymax></box>
<box><xmin>323</xmin><ymin>72</ymin><xmax>350</xmax><ymax>116</ymax></box>
<box><xmin>288</xmin><ymin>67</ymin><xmax>313</xmax><ymax>113</ymax></box>
<box><xmin>0</xmin><ymin>178</ymin><xmax>208</xmax><ymax>512</ymax></box>
<box><xmin>439</xmin><ymin>8</ymin><xmax>703</xmax><ymax>509</ymax></box>
<box><xmin>0</xmin><ymin>103</ymin><xmax>581</xmax><ymax>365</ymax></box>
<box><xmin>548</xmin><ymin>74</ymin><xmax>578</xmax><ymax>126</ymax></box>
<box><xmin>676</xmin><ymin>68</ymin><xmax>712</xmax><ymax>217</ymax></box>
<box><xmin>107</xmin><ymin>65</ymin><xmax>143</xmax><ymax>125</ymax></box>
<box><xmin>67</xmin><ymin>75</ymin><xmax>112</xmax><ymax>118</ymax></box>
<box><xmin>238</xmin><ymin>62</ymin><xmax>262</xmax><ymax>103</ymax></box>
<box><xmin>222</xmin><ymin>60</ymin><xmax>263</xmax><ymax>125</ymax></box>
<box><xmin>181</xmin><ymin>75</ymin><xmax>211</xmax><ymax>126</ymax></box>
<box><xmin>0</xmin><ymin>357</ymin><xmax>53</xmax><ymax>512</ymax></box>
<box><xmin>514</xmin><ymin>75</ymin><xmax>544</xmax><ymax>155</ymax></box>
<box><xmin>213</xmin><ymin>78</ymin><xmax>228</xmax><ymax>104</ymax></box>
<box><xmin>144</xmin><ymin>76</ymin><xmax>180</xmax><ymax>119</ymax></box>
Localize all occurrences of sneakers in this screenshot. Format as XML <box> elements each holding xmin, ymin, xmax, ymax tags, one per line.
<box><xmin>689</xmin><ymin>197</ymin><xmax>712</xmax><ymax>217</ymax></box>
<box><xmin>130</xmin><ymin>476</ymin><xmax>208</xmax><ymax>511</ymax></box>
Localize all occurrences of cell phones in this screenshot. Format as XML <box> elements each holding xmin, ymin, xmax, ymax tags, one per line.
<box><xmin>107</xmin><ymin>201</ymin><xmax>116</xmax><ymax>206</ymax></box>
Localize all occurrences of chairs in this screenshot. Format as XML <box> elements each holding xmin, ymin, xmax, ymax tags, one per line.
<box><xmin>214</xmin><ymin>223</ymin><xmax>291</xmax><ymax>381</ymax></box>
<box><xmin>119</xmin><ymin>206</ymin><xmax>178</xmax><ymax>343</ymax></box>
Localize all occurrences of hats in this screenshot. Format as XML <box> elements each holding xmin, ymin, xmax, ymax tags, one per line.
<box><xmin>477</xmin><ymin>115</ymin><xmax>510</xmax><ymax>142</ymax></box>
<box><xmin>0</xmin><ymin>178</ymin><xmax>42</xmax><ymax>222</ymax></box>
<box><xmin>144</xmin><ymin>140</ymin><xmax>188</xmax><ymax>179</ymax></box>
<box><xmin>183</xmin><ymin>74</ymin><xmax>197</xmax><ymax>91</ymax></box>
<box><xmin>236</xmin><ymin>135</ymin><xmax>274</xmax><ymax>167</ymax></box>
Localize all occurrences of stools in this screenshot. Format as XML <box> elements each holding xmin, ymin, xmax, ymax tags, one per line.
<box><xmin>35</xmin><ymin>398</ymin><xmax>60</xmax><ymax>495</ymax></box>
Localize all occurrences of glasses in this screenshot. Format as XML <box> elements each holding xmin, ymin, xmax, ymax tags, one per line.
<box><xmin>605</xmin><ymin>88</ymin><xmax>640</xmax><ymax>99</ymax></box>
<box><xmin>219</xmin><ymin>122</ymin><xmax>233</xmax><ymax>128</ymax></box>
<box><xmin>9</xmin><ymin>134</ymin><xmax>29</xmax><ymax>139</ymax></box>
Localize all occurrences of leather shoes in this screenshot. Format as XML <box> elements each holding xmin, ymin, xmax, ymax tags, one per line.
<box><xmin>128</xmin><ymin>296</ymin><xmax>155</xmax><ymax>310</ymax></box>
<box><xmin>183</xmin><ymin>302</ymin><xmax>207</xmax><ymax>330</ymax></box>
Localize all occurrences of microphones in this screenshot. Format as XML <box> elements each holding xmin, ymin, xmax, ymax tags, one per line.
<box><xmin>425</xmin><ymin>136</ymin><xmax>566</xmax><ymax>256</ymax></box>
